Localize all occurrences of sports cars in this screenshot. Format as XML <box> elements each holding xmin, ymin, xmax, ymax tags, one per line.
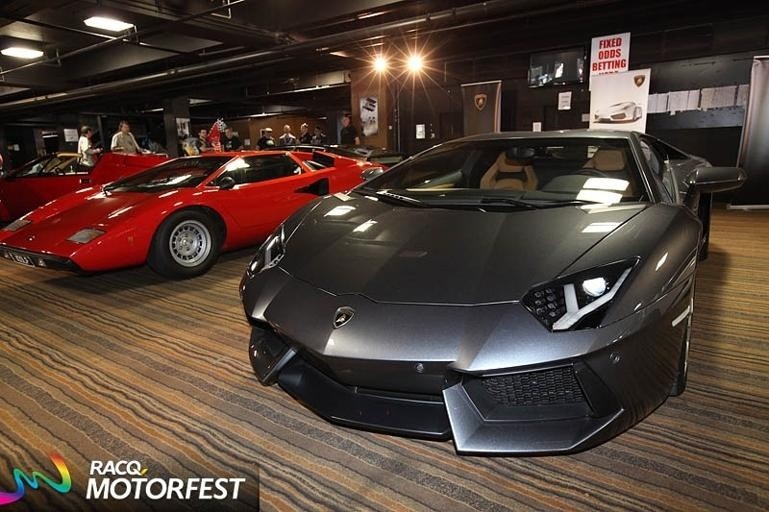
<box><xmin>594</xmin><ymin>101</ymin><xmax>642</xmax><ymax>124</ymax></box>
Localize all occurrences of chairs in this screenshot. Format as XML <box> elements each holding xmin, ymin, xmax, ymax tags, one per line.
<box><xmin>585</xmin><ymin>148</ymin><xmax>637</xmax><ymax>197</ymax></box>
<box><xmin>480</xmin><ymin>151</ymin><xmax>539</xmax><ymax>191</ymax></box>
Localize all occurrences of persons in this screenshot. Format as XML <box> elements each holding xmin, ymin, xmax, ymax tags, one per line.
<box><xmin>110</xmin><ymin>120</ymin><xmax>146</xmax><ymax>153</ymax></box>
<box><xmin>311</xmin><ymin>126</ymin><xmax>328</xmax><ymax>144</ymax></box>
<box><xmin>340</xmin><ymin>117</ymin><xmax>360</xmax><ymax>145</ymax></box>
<box><xmin>255</xmin><ymin>126</ymin><xmax>276</xmax><ymax>149</ymax></box>
<box><xmin>278</xmin><ymin>124</ymin><xmax>296</xmax><ymax>146</ymax></box>
<box><xmin>219</xmin><ymin>125</ymin><xmax>244</xmax><ymax>152</ymax></box>
<box><xmin>195</xmin><ymin>127</ymin><xmax>219</xmax><ymax>154</ymax></box>
<box><xmin>76</xmin><ymin>125</ymin><xmax>102</xmax><ymax>172</ymax></box>
<box><xmin>296</xmin><ymin>122</ymin><xmax>312</xmax><ymax>145</ymax></box>
